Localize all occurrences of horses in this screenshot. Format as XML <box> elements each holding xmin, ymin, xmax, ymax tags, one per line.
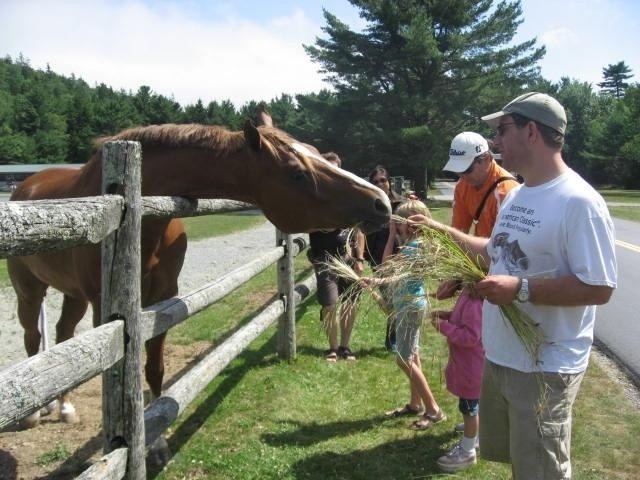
<box><xmin>6</xmin><ymin>111</ymin><xmax>391</xmax><ymax>430</ymax></box>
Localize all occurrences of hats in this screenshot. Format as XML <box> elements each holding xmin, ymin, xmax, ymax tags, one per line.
<box><xmin>481</xmin><ymin>93</ymin><xmax>567</xmax><ymax>134</ymax></box>
<box><xmin>441</xmin><ymin>132</ymin><xmax>488</xmax><ymax>172</ymax></box>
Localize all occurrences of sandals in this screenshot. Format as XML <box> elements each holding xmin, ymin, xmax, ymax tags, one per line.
<box><xmin>341</xmin><ymin>346</ymin><xmax>355</xmax><ymax>360</ymax></box>
<box><xmin>410</xmin><ymin>408</ymin><xmax>446</xmax><ymax>430</ymax></box>
<box><xmin>326</xmin><ymin>348</ymin><xmax>340</xmax><ymax>362</ymax></box>
<box><xmin>392</xmin><ymin>403</ymin><xmax>423</xmax><ymax>417</ymax></box>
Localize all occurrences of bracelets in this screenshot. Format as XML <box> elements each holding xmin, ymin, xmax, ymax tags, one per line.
<box><xmin>456</xmin><ymin>277</ymin><xmax>463</xmax><ymax>291</ymax></box>
<box><xmin>355</xmin><ymin>257</ymin><xmax>366</xmax><ymax>262</ymax></box>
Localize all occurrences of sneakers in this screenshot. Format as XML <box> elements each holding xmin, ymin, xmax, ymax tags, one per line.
<box><xmin>438</xmin><ymin>445</ymin><xmax>477</xmax><ymax>469</ymax></box>
<box><xmin>387</xmin><ymin>344</ymin><xmax>398</xmax><ymax>352</ymax></box>
<box><xmin>456</xmin><ymin>423</ymin><xmax>480</xmax><ymax>448</ymax></box>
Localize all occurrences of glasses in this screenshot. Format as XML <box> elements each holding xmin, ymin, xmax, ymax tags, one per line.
<box><xmin>496</xmin><ymin>121</ymin><xmax>519</xmax><ymax>136</ymax></box>
<box><xmin>372</xmin><ymin>178</ymin><xmax>388</xmax><ymax>185</ymax></box>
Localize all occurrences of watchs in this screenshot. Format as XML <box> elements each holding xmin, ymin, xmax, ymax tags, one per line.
<box><xmin>516</xmin><ymin>278</ymin><xmax>530</xmax><ymax>304</ymax></box>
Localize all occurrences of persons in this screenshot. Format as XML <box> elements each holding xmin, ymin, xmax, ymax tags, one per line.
<box><xmin>356</xmin><ymin>198</ymin><xmax>449</xmax><ymax>431</ymax></box>
<box><xmin>430</xmin><ymin>282</ymin><xmax>486</xmax><ymax>469</ymax></box>
<box><xmin>364</xmin><ymin>164</ymin><xmax>412</xmax><ymax>355</ymax></box>
<box><xmin>435</xmin><ymin>130</ymin><xmax>522</xmax><ymax>304</ymax></box>
<box><xmin>406</xmin><ymin>91</ymin><xmax>618</xmax><ymax>480</ymax></box>
<box><xmin>306</xmin><ymin>151</ymin><xmax>366</xmax><ymax>361</ymax></box>
